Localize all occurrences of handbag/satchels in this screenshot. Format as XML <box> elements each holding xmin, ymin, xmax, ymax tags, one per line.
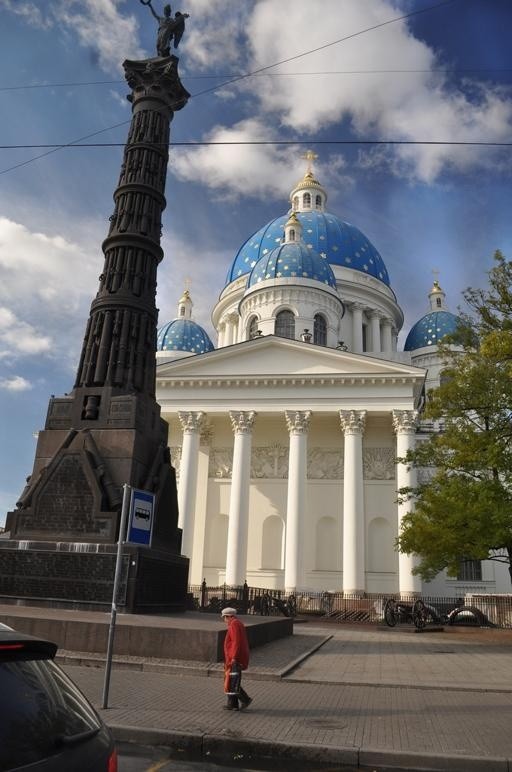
<box><xmin>224</xmin><ymin>662</ymin><xmax>242</xmax><ymax>694</ymax></box>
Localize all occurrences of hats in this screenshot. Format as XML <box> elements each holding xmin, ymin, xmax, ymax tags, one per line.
<box><xmin>221</xmin><ymin>607</ymin><xmax>237</xmax><ymax>618</ymax></box>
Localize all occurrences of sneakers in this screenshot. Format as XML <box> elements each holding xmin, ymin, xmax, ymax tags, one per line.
<box><xmin>223</xmin><ymin>697</ymin><xmax>252</xmax><ymax>711</ymax></box>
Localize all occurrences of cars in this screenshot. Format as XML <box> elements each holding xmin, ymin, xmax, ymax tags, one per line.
<box><xmin>0</xmin><ymin>621</ymin><xmax>119</xmax><ymax>772</ymax></box>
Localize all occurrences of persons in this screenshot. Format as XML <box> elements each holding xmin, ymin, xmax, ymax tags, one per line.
<box><xmin>148</xmin><ymin>3</ymin><xmax>189</xmax><ymax>57</ymax></box>
<box><xmin>217</xmin><ymin>606</ymin><xmax>253</xmax><ymax>713</ymax></box>
